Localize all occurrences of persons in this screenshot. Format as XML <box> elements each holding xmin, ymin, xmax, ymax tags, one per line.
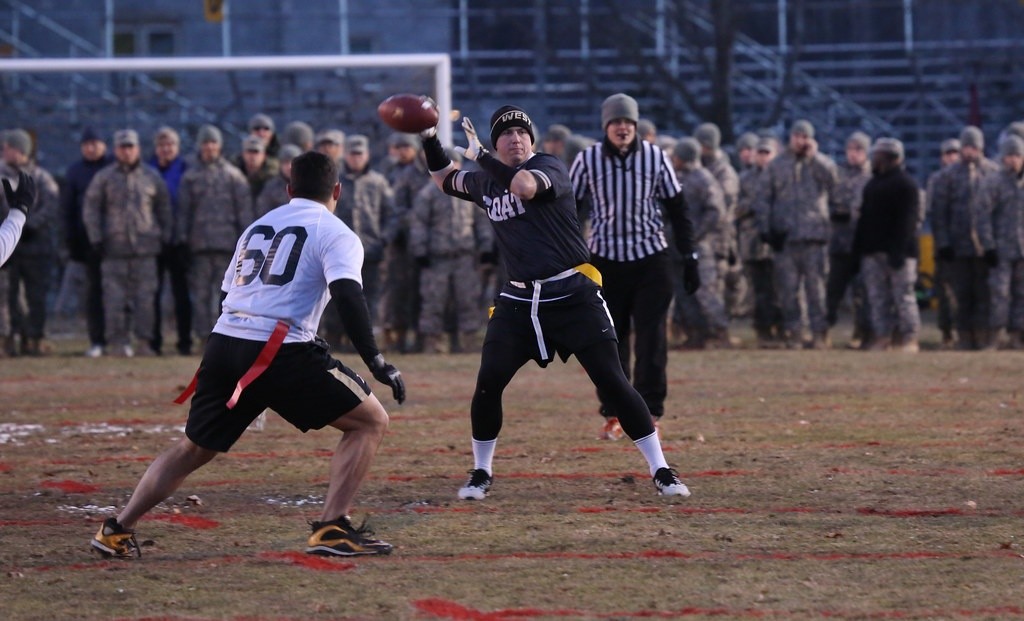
<box><xmin>91</xmin><ymin>152</ymin><xmax>406</xmax><ymax>558</ymax></box>
<box><xmin>0</xmin><ymin>113</ymin><xmax>599</xmax><ymax>359</ymax></box>
<box><xmin>569</xmin><ymin>90</ymin><xmax>700</xmax><ymax>440</ymax></box>
<box><xmin>635</xmin><ymin>119</ymin><xmax>1024</xmax><ymax>352</ymax></box>
<box><xmin>418</xmin><ymin>95</ymin><xmax>692</xmax><ymax>501</ymax></box>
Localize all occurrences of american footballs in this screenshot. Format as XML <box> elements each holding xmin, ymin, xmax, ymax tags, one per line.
<box><xmin>378</xmin><ymin>93</ymin><xmax>440</xmax><ymax>133</ymax></box>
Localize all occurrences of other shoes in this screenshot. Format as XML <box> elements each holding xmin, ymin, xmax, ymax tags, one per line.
<box><xmin>1</xmin><ymin>332</ymin><xmax>211</xmax><ymax>358</ymax></box>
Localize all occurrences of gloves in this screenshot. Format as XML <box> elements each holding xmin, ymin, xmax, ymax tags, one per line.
<box><xmin>453</xmin><ymin>115</ymin><xmax>489</xmax><ymax>162</ymax></box>
<box><xmin>416</xmin><ymin>95</ymin><xmax>440</xmax><ymax>136</ymax></box>
<box><xmin>682</xmin><ymin>256</ymin><xmax>700</xmax><ymax>295</ymax></box>
<box><xmin>367</xmin><ymin>356</ymin><xmax>407</xmax><ymax>405</ymax></box>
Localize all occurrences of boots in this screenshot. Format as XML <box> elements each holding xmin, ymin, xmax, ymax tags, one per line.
<box><xmin>664</xmin><ymin>321</ymin><xmax>1024</xmax><ymax>353</ymax></box>
<box><xmin>383</xmin><ymin>324</ymin><xmax>487</xmax><ymax>355</ymax></box>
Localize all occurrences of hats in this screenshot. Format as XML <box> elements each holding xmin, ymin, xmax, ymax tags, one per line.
<box><xmin>489</xmin><ymin>107</ymin><xmax>535</xmax><ymax>150</ymax></box>
<box><xmin>600</xmin><ymin>93</ymin><xmax>640</xmax><ymax>128</ymax></box>
<box><xmin>2</xmin><ymin>113</ymin><xmax>1024</xmax><ymax>167</ymax></box>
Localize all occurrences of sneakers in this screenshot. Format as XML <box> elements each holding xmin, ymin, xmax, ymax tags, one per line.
<box><xmin>456</xmin><ymin>466</ymin><xmax>493</xmax><ymax>501</ymax></box>
<box><xmin>91</xmin><ymin>519</ymin><xmax>142</xmax><ymax>561</ymax></box>
<box><xmin>595</xmin><ymin>415</ymin><xmax>626</xmax><ymax>441</ymax></box>
<box><xmin>653</xmin><ymin>422</ymin><xmax>662</xmax><ymax>441</ymax></box>
<box><xmin>650</xmin><ymin>467</ymin><xmax>691</xmax><ymax>498</ymax></box>
<box><xmin>305</xmin><ymin>515</ymin><xmax>393</xmax><ymax>557</ymax></box>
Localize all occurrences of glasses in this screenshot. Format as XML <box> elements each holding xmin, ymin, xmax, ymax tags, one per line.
<box><xmin>118</xmin><ymin>143</ymin><xmax>133</xmax><ymax>148</ymax></box>
<box><xmin>253</xmin><ymin>125</ymin><xmax>272</xmax><ymax>131</ymax></box>
